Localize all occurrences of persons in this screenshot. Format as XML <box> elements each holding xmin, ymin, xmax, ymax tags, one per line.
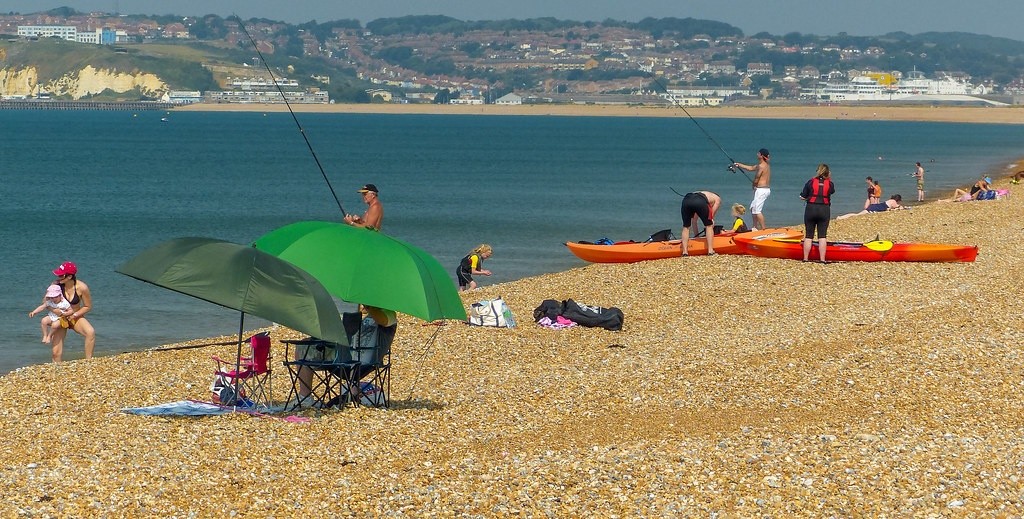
<box><xmin>681</xmin><ymin>191</ymin><xmax>721</xmax><ymax>257</ymax></box>
<box><xmin>42</xmin><ymin>261</ymin><xmax>96</xmax><ymax>362</ymax></box>
<box><xmin>29</xmin><ymin>284</ymin><xmax>75</xmax><ymax>344</ymax></box>
<box><xmin>914</xmin><ymin>162</ymin><xmax>925</xmax><ymax>201</ymax></box>
<box><xmin>725</xmin><ymin>203</ymin><xmax>748</xmax><ymax>234</ymax></box>
<box><xmin>836</xmin><ymin>194</ymin><xmax>902</xmax><ymax>221</ymax></box>
<box><xmin>803</xmin><ymin>164</ymin><xmax>835</xmax><ymax>263</ymax></box>
<box><xmin>283</xmin><ymin>305</ymin><xmax>397</xmax><ymax>408</ymax></box>
<box><xmin>456</xmin><ymin>244</ymin><xmax>495</xmax><ymax>291</ymax></box>
<box><xmin>734</xmin><ymin>148</ymin><xmax>772</xmax><ymax>230</ymax></box>
<box><xmin>864</xmin><ymin>176</ymin><xmax>878</xmax><ymax>210</ymax></box>
<box><xmin>874</xmin><ymin>181</ymin><xmax>881</xmax><ymax>204</ymax></box>
<box><xmin>938</xmin><ymin>175</ymin><xmax>992</xmax><ymax>203</ymax></box>
<box><xmin>344</xmin><ymin>184</ymin><xmax>383</xmax><ymax>230</ymax></box>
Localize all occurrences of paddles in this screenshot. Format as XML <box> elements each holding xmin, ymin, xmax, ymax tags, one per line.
<box><xmin>772</xmin><ymin>237</ymin><xmax>893</xmax><ymax>251</ymax></box>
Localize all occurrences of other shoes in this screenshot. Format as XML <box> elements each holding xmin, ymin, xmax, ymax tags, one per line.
<box><xmin>287</xmin><ymin>395</ymin><xmax>313</xmax><ymax>407</ymax></box>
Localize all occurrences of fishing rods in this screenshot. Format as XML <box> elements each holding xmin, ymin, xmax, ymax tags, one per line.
<box><xmin>635</xmin><ymin>61</ymin><xmax>753</xmax><ymax>183</ymax></box>
<box><xmin>890</xmin><ymin>170</ymin><xmax>929</xmax><ymax>178</ymax></box>
<box><xmin>233</xmin><ymin>14</ymin><xmax>346</xmax><ymax>218</ymax></box>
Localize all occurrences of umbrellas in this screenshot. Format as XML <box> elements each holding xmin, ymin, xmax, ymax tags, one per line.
<box><xmin>247</xmin><ymin>221</ymin><xmax>468</xmax><ymax>322</ymax></box>
<box><xmin>114</xmin><ymin>237</ymin><xmax>350</xmax><ymax>410</ymax></box>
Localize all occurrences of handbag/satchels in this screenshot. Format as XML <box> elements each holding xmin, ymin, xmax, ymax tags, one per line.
<box><xmin>208</xmin><ymin>367</ymin><xmax>246</xmax><ymax>403</ymax></box>
<box><xmin>470</xmin><ymin>299</ymin><xmax>507</xmax><ymax>327</ymax></box>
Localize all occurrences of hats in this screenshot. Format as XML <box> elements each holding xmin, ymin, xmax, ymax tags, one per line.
<box><xmin>357</xmin><ymin>184</ymin><xmax>378</xmax><ymax>193</ymax></box>
<box><xmin>759</xmin><ymin>149</ymin><xmax>770</xmax><ymax>159</ymax></box>
<box><xmin>985</xmin><ymin>178</ymin><xmax>991</xmax><ymax>184</ymax></box>
<box><xmin>52</xmin><ymin>262</ymin><xmax>77</xmax><ymax>276</ymax></box>
<box><xmin>45</xmin><ymin>285</ymin><xmax>62</xmax><ymax>298</ymax></box>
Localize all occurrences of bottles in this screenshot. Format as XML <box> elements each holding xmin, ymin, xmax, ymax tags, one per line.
<box><xmin>501</xmin><ymin>303</ymin><xmax>516</xmax><ymax>328</ymax></box>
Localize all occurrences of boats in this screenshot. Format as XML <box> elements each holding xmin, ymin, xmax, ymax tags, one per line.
<box><xmin>561</xmin><ymin>226</ymin><xmax>805</xmax><ymax>264</ymax></box>
<box><xmin>728</xmin><ymin>235</ymin><xmax>979</xmax><ymax>262</ymax></box>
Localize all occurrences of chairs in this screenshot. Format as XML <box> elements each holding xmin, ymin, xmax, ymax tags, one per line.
<box><xmin>279</xmin><ymin>311</ymin><xmax>396</xmax><ymax>413</ymax></box>
<box><xmin>210</xmin><ymin>335</ymin><xmax>272</xmax><ymax>412</ymax></box>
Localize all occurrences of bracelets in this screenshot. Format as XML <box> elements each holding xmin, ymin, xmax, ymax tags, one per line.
<box><xmin>351</xmin><ymin>222</ymin><xmax>354</xmax><ymax>226</ymax></box>
<box><xmin>73</xmin><ymin>313</ymin><xmax>79</xmax><ymax>321</ymax></box>
